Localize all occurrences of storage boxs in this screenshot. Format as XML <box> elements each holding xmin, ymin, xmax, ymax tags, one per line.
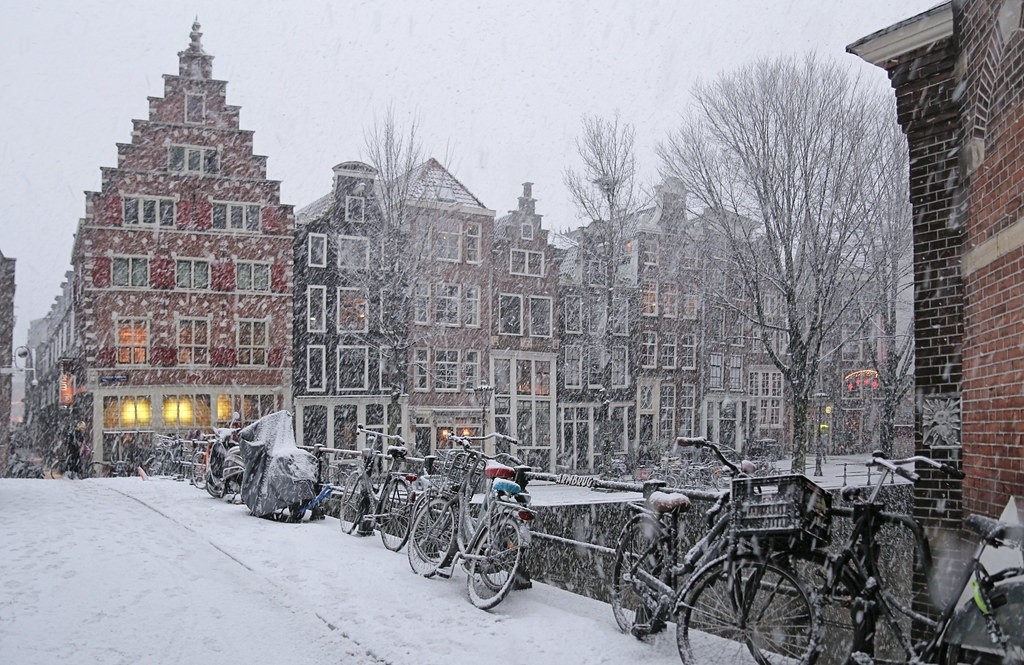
<box><xmin>730</xmin><ymin>475</ymin><xmax>833</xmax><ymax>547</ymax></box>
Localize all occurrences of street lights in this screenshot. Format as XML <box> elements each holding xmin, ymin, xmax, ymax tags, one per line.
<box><xmin>814</xmin><ymin>390</ymin><xmax>829</xmax><ymax>477</ymax></box>
<box><xmin>474</xmin><ymin>381</ymin><xmax>494</xmax><ymax>453</ymax></box>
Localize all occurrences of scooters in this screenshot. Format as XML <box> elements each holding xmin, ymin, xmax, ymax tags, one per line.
<box><xmin>206</xmin><ymin>410</ymin><xmax>316</xmax><ymax>519</ymax></box>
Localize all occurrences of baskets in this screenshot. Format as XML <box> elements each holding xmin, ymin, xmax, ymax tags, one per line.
<box><xmin>730</xmin><ymin>473</ymin><xmax>833</xmax><ymax>551</ymax></box>
<box><xmin>429</xmin><ymin>449</ymin><xmax>487</xmax><ymax>501</ymax></box>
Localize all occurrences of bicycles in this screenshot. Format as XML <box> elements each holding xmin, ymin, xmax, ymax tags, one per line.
<box><xmin>612</xmin><ymin>436</ymin><xmax>1024</xmax><ymax>665</ymax></box>
<box><xmin>4</xmin><ymin>433</ymin><xmax>207</xmax><ymax>489</ymax></box>
<box><xmin>340</xmin><ymin>425</ymin><xmax>537</xmax><ymax>609</ymax></box>
